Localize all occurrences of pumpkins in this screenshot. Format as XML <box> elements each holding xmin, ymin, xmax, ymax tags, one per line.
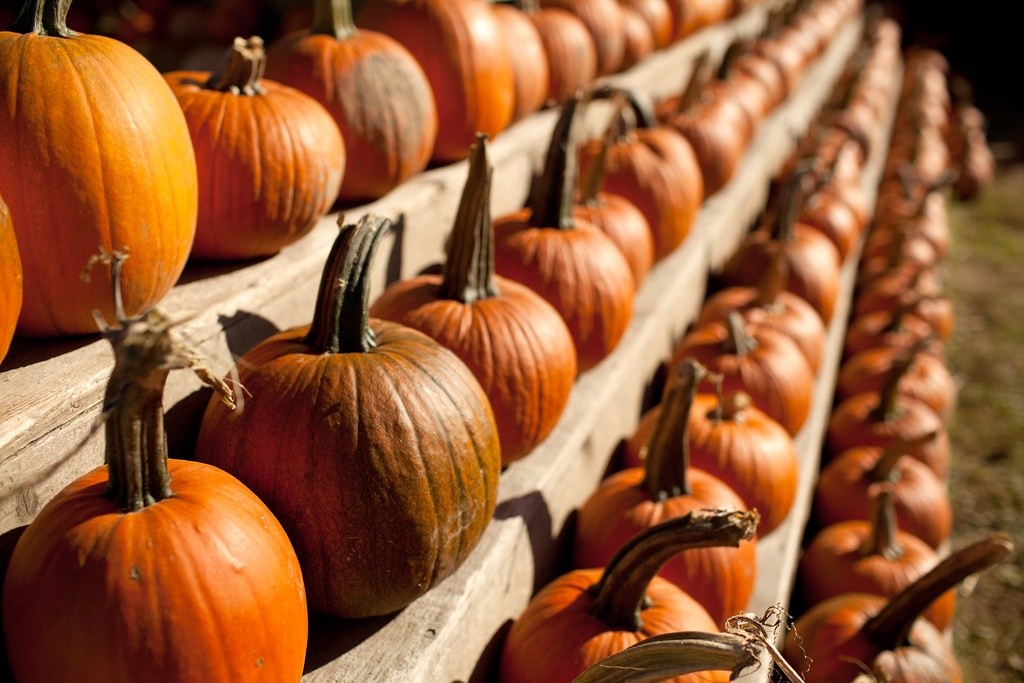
<box><xmin>0</xmin><ymin>0</ymin><xmax>1015</xmax><ymax>683</ymax></box>
